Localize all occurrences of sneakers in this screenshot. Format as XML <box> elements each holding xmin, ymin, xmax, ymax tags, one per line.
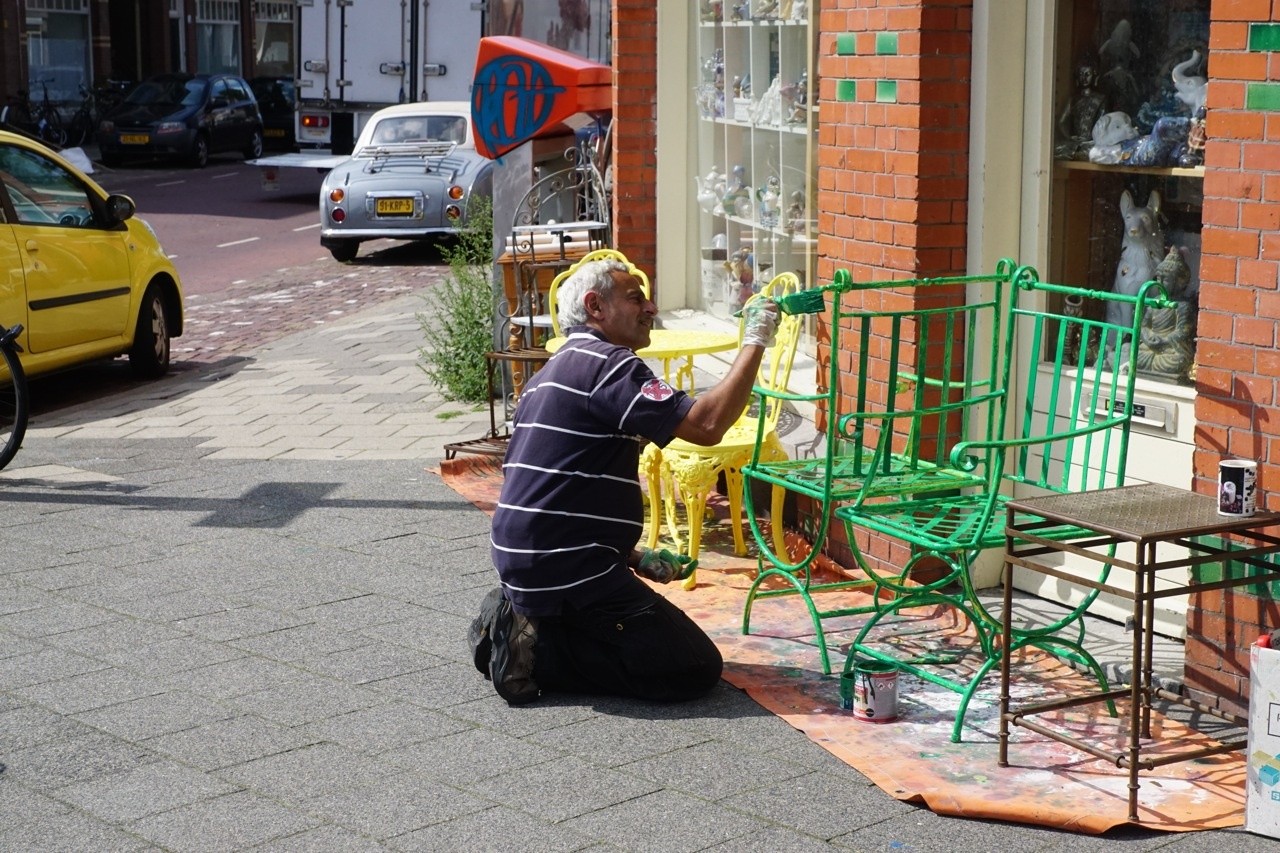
<box><xmin>464</xmin><ymin>587</ymin><xmax>510</xmax><ymax>677</ymax></box>
<box><xmin>490</xmin><ymin>598</ymin><xmax>539</xmax><ymax>703</ymax></box>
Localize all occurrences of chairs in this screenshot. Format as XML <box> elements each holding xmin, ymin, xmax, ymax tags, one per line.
<box><xmin>647</xmin><ymin>272</ymin><xmax>800</xmax><ymax>590</ymax></box>
<box><xmin>550</xmin><ymin>251</ymin><xmax>650</xmax><ymax>339</ymax></box>
<box><xmin>743</xmin><ymin>259</ymin><xmax>1177</xmax><ymax>746</ymax></box>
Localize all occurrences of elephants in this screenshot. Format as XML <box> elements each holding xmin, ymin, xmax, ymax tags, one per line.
<box><xmin>1171</xmin><ymin>49</ymin><xmax>1208</xmax><ymax>119</ymax></box>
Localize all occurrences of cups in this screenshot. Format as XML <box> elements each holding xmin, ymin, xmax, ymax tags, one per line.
<box><xmin>1217</xmin><ymin>459</ymin><xmax>1258</xmax><ymax>517</ymax></box>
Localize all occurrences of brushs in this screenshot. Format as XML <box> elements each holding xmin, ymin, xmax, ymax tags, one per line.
<box><xmin>732</xmin><ymin>286</ymin><xmax>825</xmax><ymax>320</ymax></box>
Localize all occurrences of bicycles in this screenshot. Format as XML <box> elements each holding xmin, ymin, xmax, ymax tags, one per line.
<box><xmin>65</xmin><ymin>83</ymin><xmax>99</xmax><ymax>147</ymax></box>
<box><xmin>0</xmin><ymin>78</ymin><xmax>64</xmax><ymax>147</ymax></box>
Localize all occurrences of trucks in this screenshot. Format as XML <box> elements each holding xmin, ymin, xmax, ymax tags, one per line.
<box><xmin>243</xmin><ymin>0</ymin><xmax>613</xmax><ymax>172</ymax></box>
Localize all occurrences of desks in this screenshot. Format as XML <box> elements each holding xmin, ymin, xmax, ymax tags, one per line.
<box><xmin>997</xmin><ymin>481</ymin><xmax>1280</xmax><ymax>814</ymax></box>
<box><xmin>635</xmin><ymin>331</ymin><xmax>738</xmax><ymax>402</ymax></box>
<box><xmin>496</xmin><ymin>238</ymin><xmax>607</xmax><ymax>396</ymax></box>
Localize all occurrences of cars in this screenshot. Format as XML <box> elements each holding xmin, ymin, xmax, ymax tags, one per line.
<box><xmin>318</xmin><ymin>101</ymin><xmax>492</xmax><ymax>262</ymax></box>
<box><xmin>0</xmin><ymin>127</ymin><xmax>184</xmax><ymax>397</ymax></box>
<box><xmin>246</xmin><ymin>74</ymin><xmax>295</xmax><ymax>151</ymax></box>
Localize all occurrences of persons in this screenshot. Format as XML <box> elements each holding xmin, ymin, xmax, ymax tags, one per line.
<box><xmin>1054</xmin><ymin>64</ymin><xmax>1108</xmax><ymax>161</ymax></box>
<box><xmin>1133</xmin><ymin>245</ymin><xmax>1199</xmax><ymax>372</ymax></box>
<box><xmin>251</xmin><ymin>75</ymin><xmax>290</xmax><ymax>191</ymax></box>
<box><xmin>472</xmin><ymin>259</ymin><xmax>781</xmax><ymax>707</ymax></box>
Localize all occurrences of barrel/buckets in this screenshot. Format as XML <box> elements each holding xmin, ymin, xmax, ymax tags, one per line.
<box><xmin>853</xmin><ymin>659</ymin><xmax>899</xmax><ymax>724</ymax></box>
<box><xmin>840</xmin><ymin>671</ymin><xmax>854</xmax><ymax>710</ymax></box>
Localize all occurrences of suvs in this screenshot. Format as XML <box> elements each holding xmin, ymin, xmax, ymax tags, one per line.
<box><xmin>89</xmin><ymin>69</ymin><xmax>263</xmax><ymax>172</ymax></box>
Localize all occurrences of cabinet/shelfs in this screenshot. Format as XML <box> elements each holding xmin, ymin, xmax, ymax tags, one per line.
<box><xmin>692</xmin><ymin>1</ymin><xmax>816</xmax><ymax>342</ymax></box>
<box><xmin>1048</xmin><ymin>1</ymin><xmax>1206</xmax><ymax>386</ymax></box>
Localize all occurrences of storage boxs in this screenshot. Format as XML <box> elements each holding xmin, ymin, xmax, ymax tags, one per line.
<box><xmin>1247</xmin><ymin>639</ymin><xmax>1280</xmax><ymax>841</ymax></box>
